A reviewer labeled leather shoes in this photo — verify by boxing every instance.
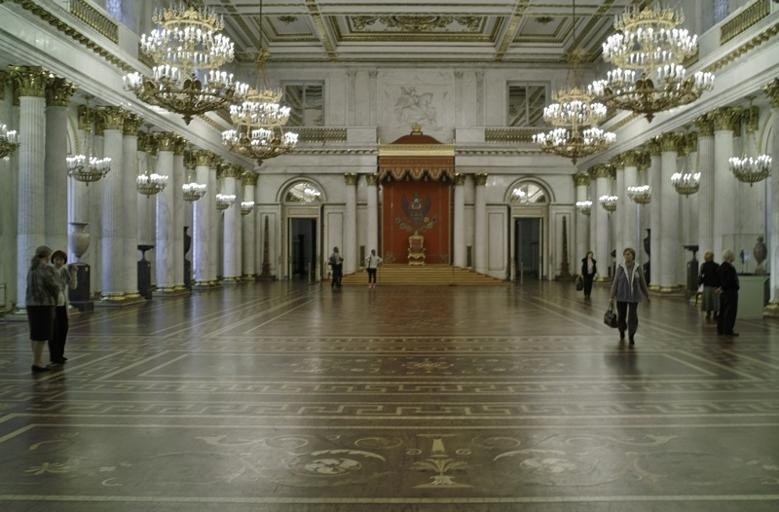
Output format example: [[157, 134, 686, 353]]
[[725, 332, 739, 336], [32, 356, 67, 372]]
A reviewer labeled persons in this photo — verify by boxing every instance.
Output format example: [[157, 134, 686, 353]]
[[328, 246, 344, 288], [44, 250, 79, 364], [25, 245, 63, 371], [363, 249, 383, 288], [608, 247, 653, 347], [713, 247, 740, 336], [580, 250, 597, 299], [697, 250, 721, 322]]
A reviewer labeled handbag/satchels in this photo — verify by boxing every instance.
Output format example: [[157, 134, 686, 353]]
[[603, 303, 617, 328], [575, 275, 584, 291]]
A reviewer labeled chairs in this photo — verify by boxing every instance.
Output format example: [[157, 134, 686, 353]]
[[406, 235, 426, 266]]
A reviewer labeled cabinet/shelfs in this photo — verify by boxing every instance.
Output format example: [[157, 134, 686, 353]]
[[735, 272, 770, 321]]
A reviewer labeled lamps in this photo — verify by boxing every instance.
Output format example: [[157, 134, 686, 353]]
[[0, 121, 24, 162], [121, 0, 300, 167], [531, 1, 717, 168], [64, 94, 256, 217], [573, 94, 772, 219]]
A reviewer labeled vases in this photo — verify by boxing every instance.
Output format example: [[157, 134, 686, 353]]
[[184, 226, 191, 256], [754, 237, 767, 265], [69, 221, 90, 265]]
